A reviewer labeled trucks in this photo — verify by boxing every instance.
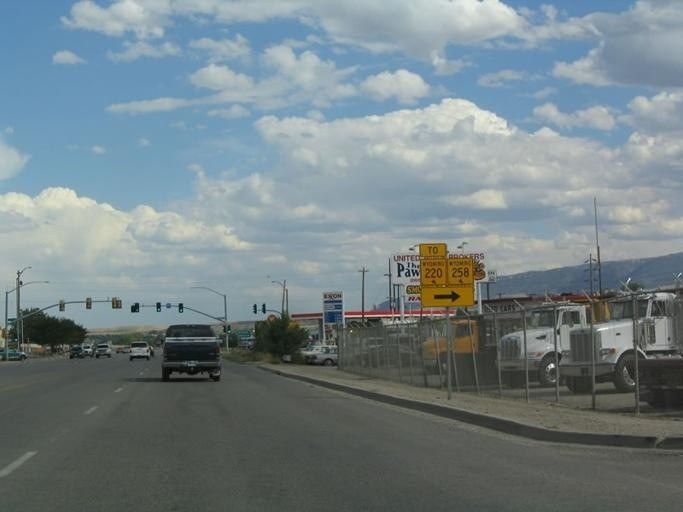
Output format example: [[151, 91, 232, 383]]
[[415, 310, 530, 374], [558, 291, 682, 393], [493, 302, 609, 387]]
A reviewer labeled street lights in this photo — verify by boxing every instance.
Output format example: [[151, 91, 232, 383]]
[[4, 280, 50, 362], [156, 302, 160, 312], [408, 243, 418, 250], [15, 266, 31, 355], [456, 240, 468, 254], [178, 302, 183, 313], [271, 280, 288, 322], [189, 286, 228, 351], [134, 302, 138, 312]]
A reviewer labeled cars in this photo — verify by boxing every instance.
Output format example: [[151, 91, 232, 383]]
[[237, 330, 419, 366], [68, 338, 154, 361], [0, 348, 29, 361]]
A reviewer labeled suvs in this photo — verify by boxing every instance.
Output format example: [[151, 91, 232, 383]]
[[161, 324, 220, 383]]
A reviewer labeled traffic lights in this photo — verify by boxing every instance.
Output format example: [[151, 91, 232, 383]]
[[227, 324, 229, 334], [262, 303, 265, 314], [253, 304, 256, 314], [222, 324, 225, 333]]
